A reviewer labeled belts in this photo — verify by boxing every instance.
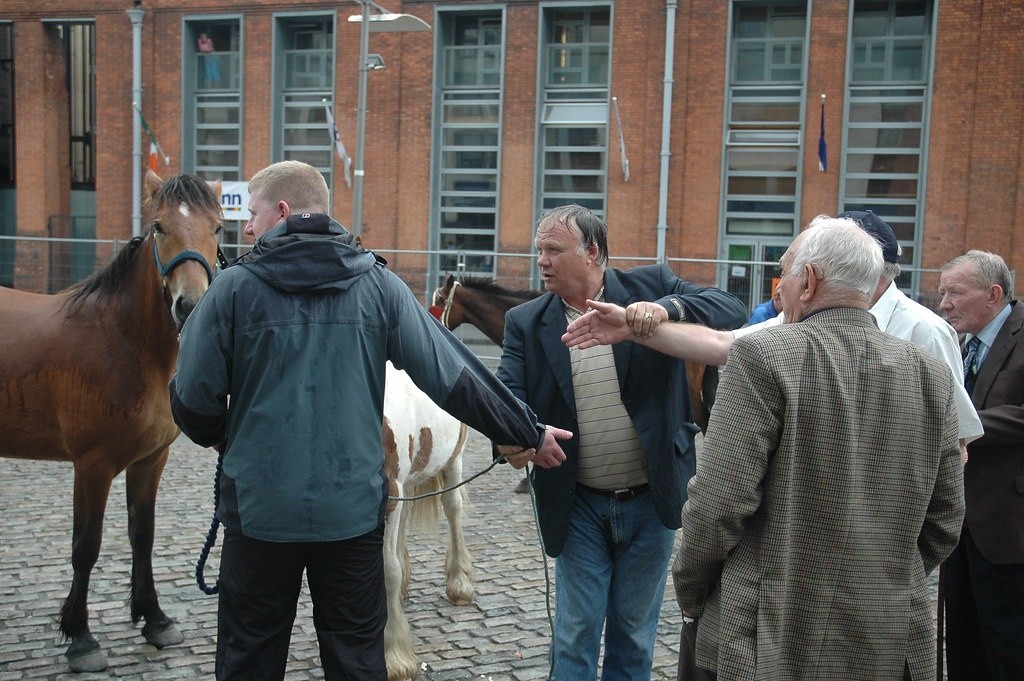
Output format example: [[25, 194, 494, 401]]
[[578, 482, 651, 502]]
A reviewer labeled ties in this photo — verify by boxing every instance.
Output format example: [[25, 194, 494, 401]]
[[963, 336, 982, 390]]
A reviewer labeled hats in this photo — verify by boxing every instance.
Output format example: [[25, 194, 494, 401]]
[[834, 211, 902, 263]]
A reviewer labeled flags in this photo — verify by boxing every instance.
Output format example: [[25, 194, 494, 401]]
[[139, 111, 159, 172], [322, 105, 352, 191], [613, 102, 628, 184], [817, 103, 829, 175]]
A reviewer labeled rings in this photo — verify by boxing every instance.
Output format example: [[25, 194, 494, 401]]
[[644, 313, 653, 319]]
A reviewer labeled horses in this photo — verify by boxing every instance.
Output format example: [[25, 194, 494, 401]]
[[0, 168, 224, 673], [381, 353, 474, 681], [427, 269, 721, 494]]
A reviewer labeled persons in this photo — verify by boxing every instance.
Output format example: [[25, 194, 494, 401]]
[[198, 32, 223, 88], [939, 247, 1024, 681], [492, 200, 749, 681], [167, 158, 573, 681], [561, 209, 985, 681]]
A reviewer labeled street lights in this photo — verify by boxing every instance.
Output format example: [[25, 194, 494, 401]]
[[343, 0, 431, 242]]
